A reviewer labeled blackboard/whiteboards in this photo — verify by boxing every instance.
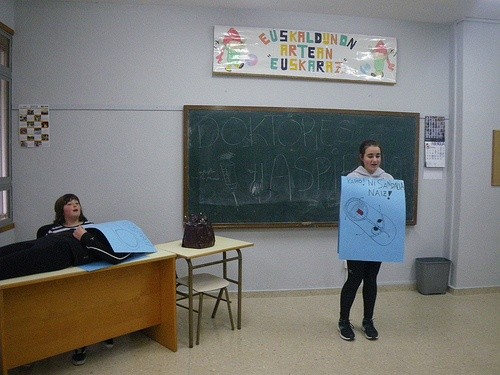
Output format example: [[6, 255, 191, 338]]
[[182, 105, 420, 229]]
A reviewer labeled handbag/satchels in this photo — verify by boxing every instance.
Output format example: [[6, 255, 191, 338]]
[[182, 213, 215, 249]]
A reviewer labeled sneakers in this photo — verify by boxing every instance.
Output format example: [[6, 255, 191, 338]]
[[103, 339, 114, 348], [361, 318, 379, 340], [336, 318, 356, 340], [72, 347, 87, 365]]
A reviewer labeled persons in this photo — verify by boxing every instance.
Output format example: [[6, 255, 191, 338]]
[[336, 140, 394, 341], [44, 194, 114, 364]]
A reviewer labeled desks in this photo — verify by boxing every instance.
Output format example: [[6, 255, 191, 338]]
[[154, 235, 254, 348], [0, 246, 177, 375]]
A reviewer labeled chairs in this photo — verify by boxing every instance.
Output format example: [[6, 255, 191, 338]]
[[176, 273, 234, 345]]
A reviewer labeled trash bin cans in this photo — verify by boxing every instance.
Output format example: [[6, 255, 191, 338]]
[[415, 257, 451, 295]]
